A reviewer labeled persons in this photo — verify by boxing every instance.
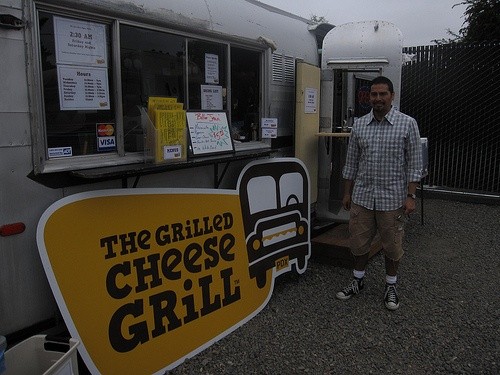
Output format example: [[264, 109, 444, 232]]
[[336, 75, 423, 309]]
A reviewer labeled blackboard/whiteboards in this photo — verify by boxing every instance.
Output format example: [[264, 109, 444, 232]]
[[185, 109, 236, 158]]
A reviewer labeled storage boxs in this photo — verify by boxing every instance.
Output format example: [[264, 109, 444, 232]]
[[5, 335, 80, 375]]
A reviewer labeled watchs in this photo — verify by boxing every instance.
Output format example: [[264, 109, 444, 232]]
[[408, 193, 416, 199]]
